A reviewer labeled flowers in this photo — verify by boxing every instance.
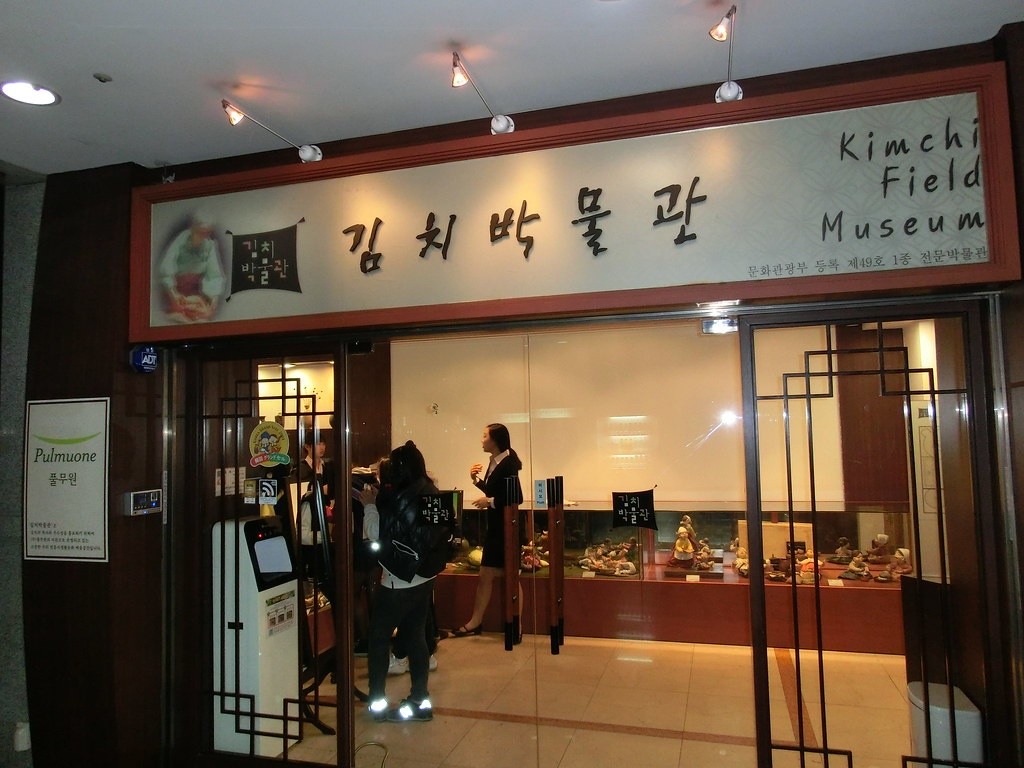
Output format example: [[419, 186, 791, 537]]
[[291, 384, 324, 404]]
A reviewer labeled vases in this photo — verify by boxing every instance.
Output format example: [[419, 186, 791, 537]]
[[303, 404, 311, 429]]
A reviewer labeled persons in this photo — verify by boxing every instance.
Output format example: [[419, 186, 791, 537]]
[[845, 550, 869, 578], [578, 537, 639, 576], [521, 527, 549, 573], [783, 545, 825, 584], [451, 424, 523, 644], [834, 533, 890, 564], [290, 431, 455, 722], [878, 548, 913, 580], [666, 515, 715, 570], [734, 546, 749, 575]]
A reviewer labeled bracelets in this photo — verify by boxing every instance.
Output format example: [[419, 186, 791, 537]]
[[486, 498, 490, 506]]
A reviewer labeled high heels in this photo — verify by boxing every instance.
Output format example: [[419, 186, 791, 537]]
[[451, 623, 482, 637], [519, 630, 522, 642]]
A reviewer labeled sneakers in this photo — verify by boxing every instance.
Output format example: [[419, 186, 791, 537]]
[[429, 654, 437, 671], [387, 698, 433, 721], [388, 658, 410, 673], [367, 696, 388, 722]]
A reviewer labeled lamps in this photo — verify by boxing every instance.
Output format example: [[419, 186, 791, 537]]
[[220, 98, 322, 164], [451, 51, 515, 134], [709, 4, 743, 103]]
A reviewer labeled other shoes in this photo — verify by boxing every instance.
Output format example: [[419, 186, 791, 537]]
[[433, 630, 448, 639]]
